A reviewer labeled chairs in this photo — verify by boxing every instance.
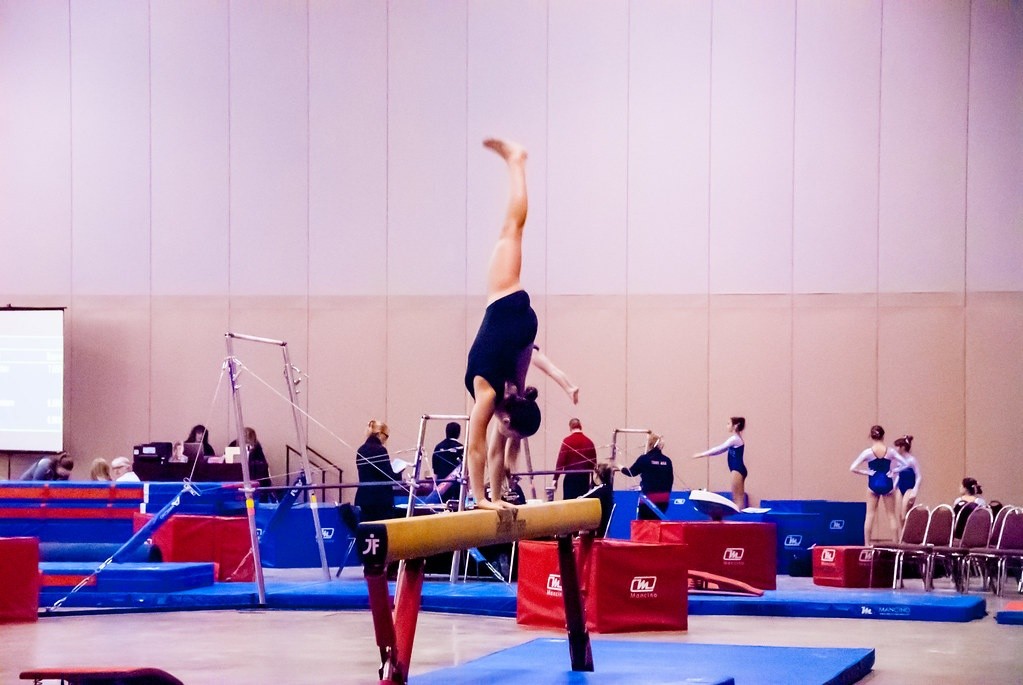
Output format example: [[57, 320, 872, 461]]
[[336, 502, 368, 577], [869, 498, 1023, 598]]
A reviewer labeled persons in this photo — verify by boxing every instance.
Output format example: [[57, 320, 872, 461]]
[[505, 345, 578, 470], [851, 425, 908, 547], [110, 457, 140, 482], [551, 418, 597, 501], [953, 478, 984, 507], [243, 427, 272, 504], [186, 425, 215, 456], [355, 420, 404, 521], [613, 434, 672, 521], [893, 436, 921, 539], [576, 464, 612, 537], [21, 452, 73, 481], [692, 417, 747, 511], [464, 139, 537, 510], [431, 422, 469, 505], [167, 441, 188, 463], [89, 458, 110, 481]]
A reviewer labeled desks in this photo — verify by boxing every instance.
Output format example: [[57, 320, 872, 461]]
[[387, 504, 511, 578]]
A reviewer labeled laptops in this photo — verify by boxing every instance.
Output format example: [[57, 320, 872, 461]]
[[225, 447, 248, 463], [183, 443, 206, 464]]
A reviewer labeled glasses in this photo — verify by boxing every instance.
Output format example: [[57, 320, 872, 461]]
[[381, 431, 389, 438], [112, 466, 124, 471]]
